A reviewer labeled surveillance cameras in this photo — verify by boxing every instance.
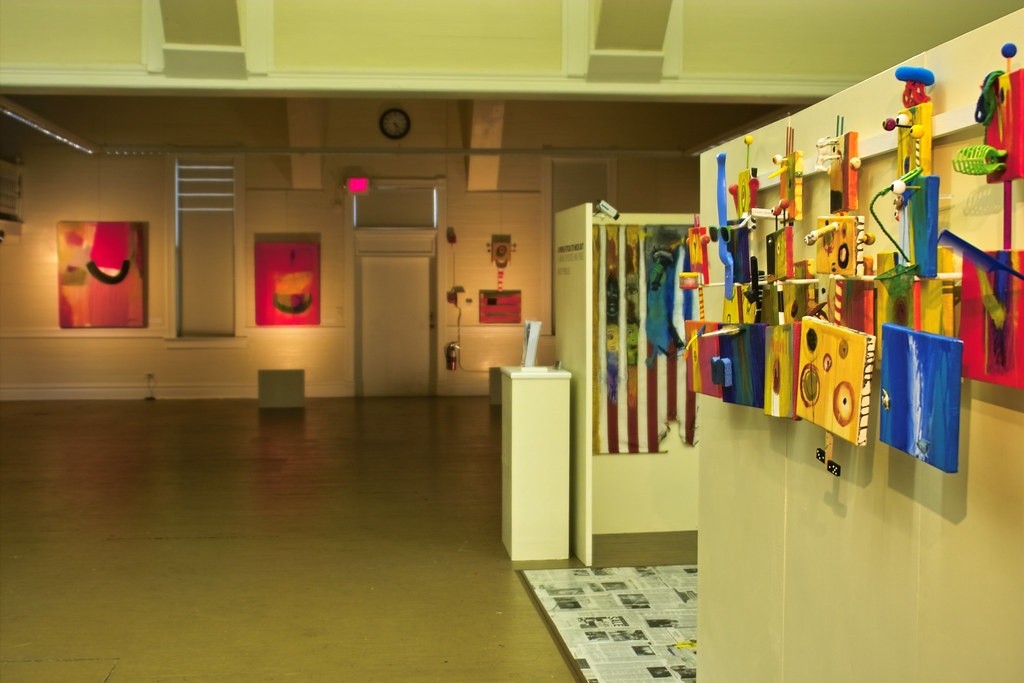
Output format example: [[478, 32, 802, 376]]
[[597, 200, 620, 220]]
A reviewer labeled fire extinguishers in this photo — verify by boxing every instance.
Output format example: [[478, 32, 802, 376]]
[[447, 341, 460, 370]]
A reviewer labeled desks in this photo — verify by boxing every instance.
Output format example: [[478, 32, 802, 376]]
[[499, 364, 573, 570]]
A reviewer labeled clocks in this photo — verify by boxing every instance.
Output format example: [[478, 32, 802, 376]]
[[379, 108, 410, 139]]
